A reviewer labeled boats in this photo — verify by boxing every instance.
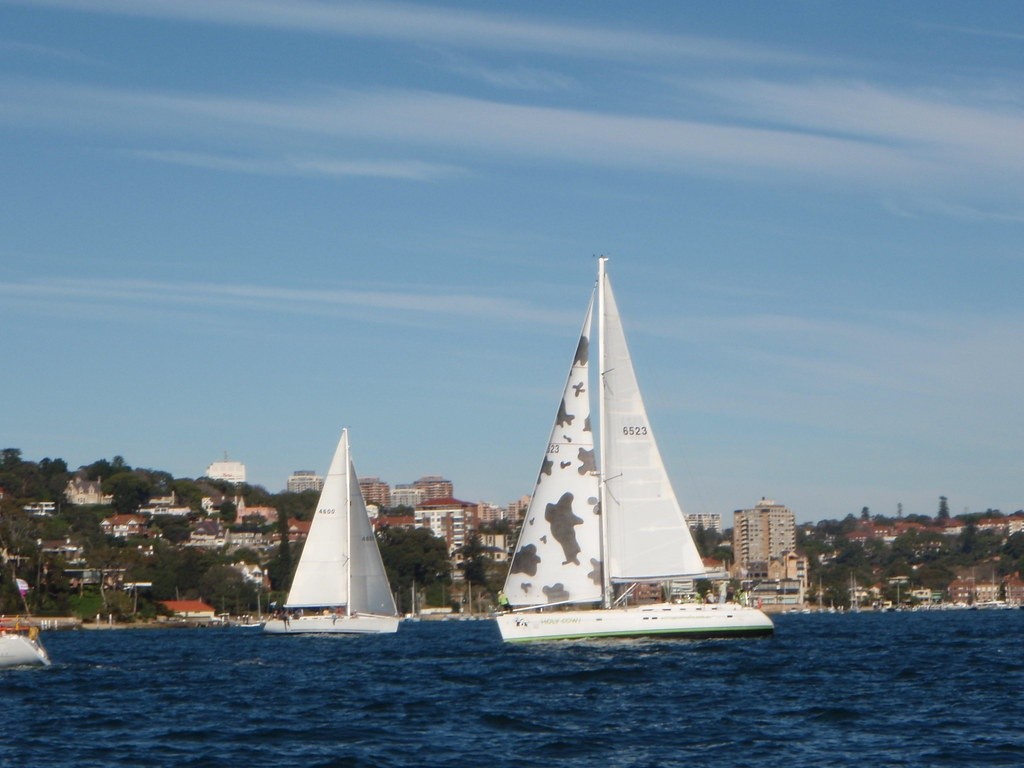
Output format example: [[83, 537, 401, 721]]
[[0, 532, 51, 671]]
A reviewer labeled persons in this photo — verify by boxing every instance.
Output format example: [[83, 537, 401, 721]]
[[498, 591, 509, 611], [273, 607, 357, 631], [705, 587, 743, 605]]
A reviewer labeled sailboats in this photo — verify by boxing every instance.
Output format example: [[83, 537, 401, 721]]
[[488, 252, 774, 643], [262, 427, 401, 634], [780, 566, 1024, 614], [408, 578, 489, 621]]
